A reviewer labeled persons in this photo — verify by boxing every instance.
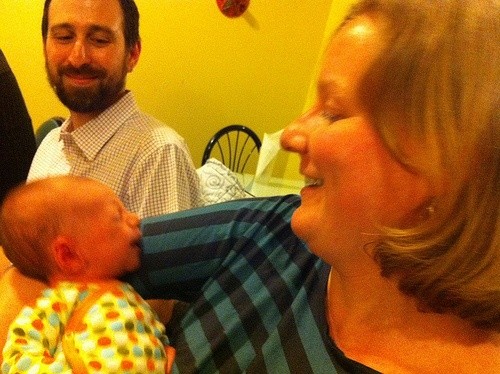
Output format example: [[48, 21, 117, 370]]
[[0, 0, 500, 374], [25, 0, 203, 219], [0, 177, 177, 374]]
